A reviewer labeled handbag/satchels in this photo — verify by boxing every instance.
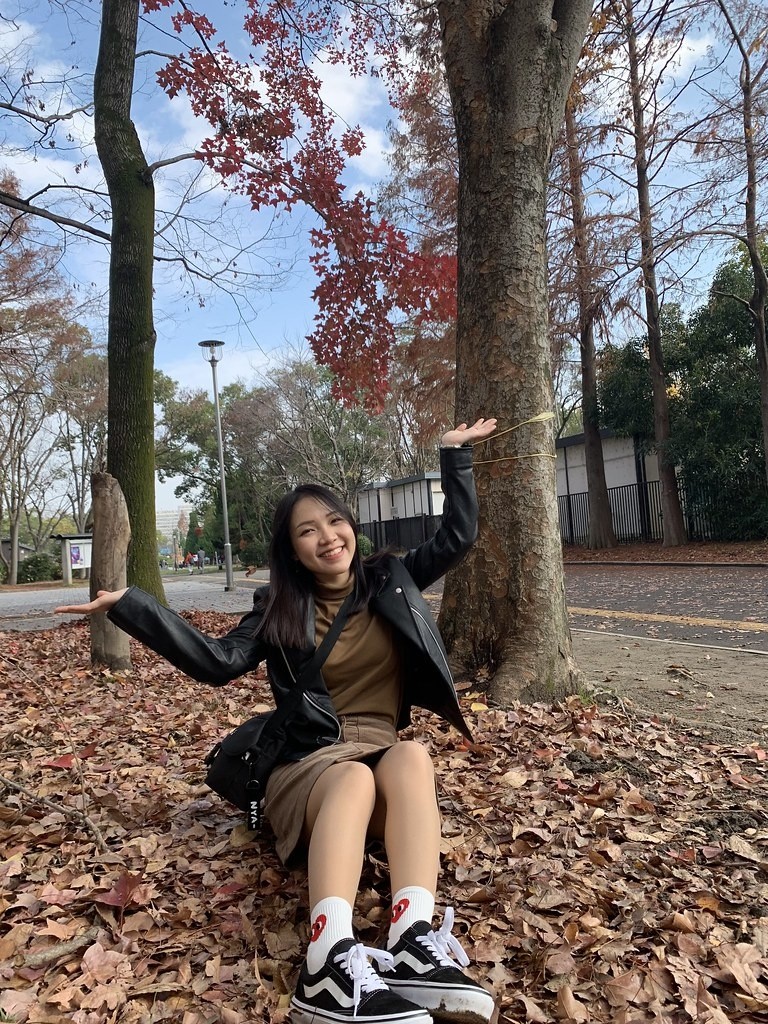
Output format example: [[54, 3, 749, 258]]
[[203, 706, 296, 831]]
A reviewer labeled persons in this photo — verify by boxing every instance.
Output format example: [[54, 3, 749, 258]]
[[197, 547, 205, 574], [54, 418, 497, 1024], [185, 551, 193, 575]]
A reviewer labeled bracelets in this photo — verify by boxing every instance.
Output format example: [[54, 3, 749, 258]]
[[440, 445, 460, 448]]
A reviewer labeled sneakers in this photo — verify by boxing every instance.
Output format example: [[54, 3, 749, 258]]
[[371, 906, 495, 1024], [289, 938, 433, 1024]]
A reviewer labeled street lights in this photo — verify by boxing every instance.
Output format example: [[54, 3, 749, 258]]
[[198, 340, 235, 592]]
